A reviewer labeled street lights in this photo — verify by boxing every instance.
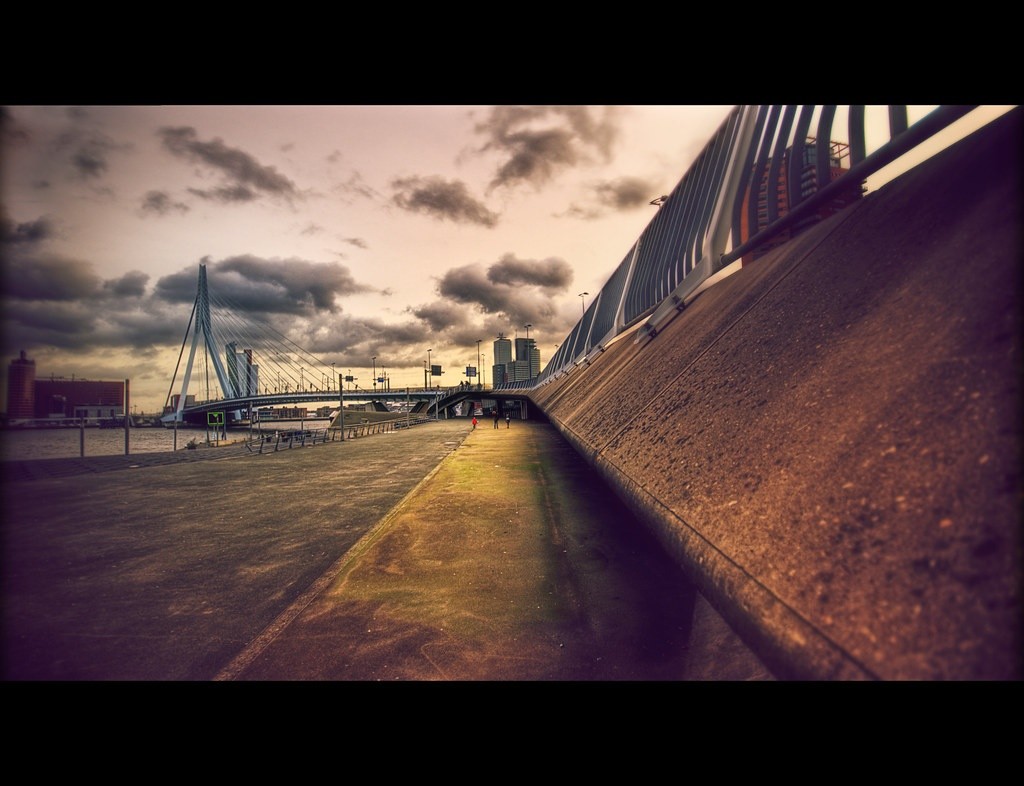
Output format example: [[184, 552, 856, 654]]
[[476, 339, 486, 391], [257, 367, 306, 392], [329, 363, 336, 392], [424, 349, 434, 390], [372, 356, 378, 391], [580, 292, 589, 313], [523, 323, 532, 379]]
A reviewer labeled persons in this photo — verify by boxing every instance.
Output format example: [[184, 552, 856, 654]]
[[505, 412, 510, 428], [472, 416, 478, 429], [493, 405, 499, 429]]
